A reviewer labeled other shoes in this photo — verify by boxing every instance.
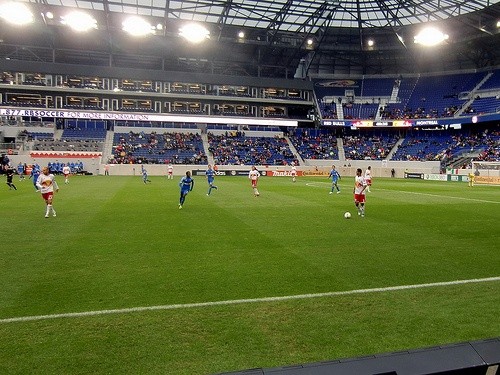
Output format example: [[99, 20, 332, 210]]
[[329, 192, 332, 194], [215, 186, 218, 190], [337, 191, 340, 194], [255, 192, 259, 196], [357, 211, 365, 218], [178, 204, 182, 210], [206, 194, 209, 196]]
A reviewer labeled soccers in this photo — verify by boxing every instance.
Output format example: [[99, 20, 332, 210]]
[[345, 212, 351, 218]]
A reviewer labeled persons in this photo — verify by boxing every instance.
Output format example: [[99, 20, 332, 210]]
[[35, 126, 101, 153], [353, 169, 367, 217], [179, 171, 194, 209], [328, 165, 342, 194], [3, 148, 17, 190], [365, 166, 372, 192], [167, 164, 173, 179], [37, 166, 59, 218], [140, 165, 151, 184], [322, 105, 474, 119], [286, 129, 500, 178], [105, 164, 109, 175], [249, 166, 260, 195], [467, 172, 475, 187], [18, 162, 40, 191], [48, 161, 83, 183], [108, 130, 299, 166], [291, 168, 296, 181], [206, 164, 218, 196]]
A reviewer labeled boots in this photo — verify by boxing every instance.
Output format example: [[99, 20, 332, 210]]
[[51, 205, 56, 218], [44, 205, 52, 217]]
